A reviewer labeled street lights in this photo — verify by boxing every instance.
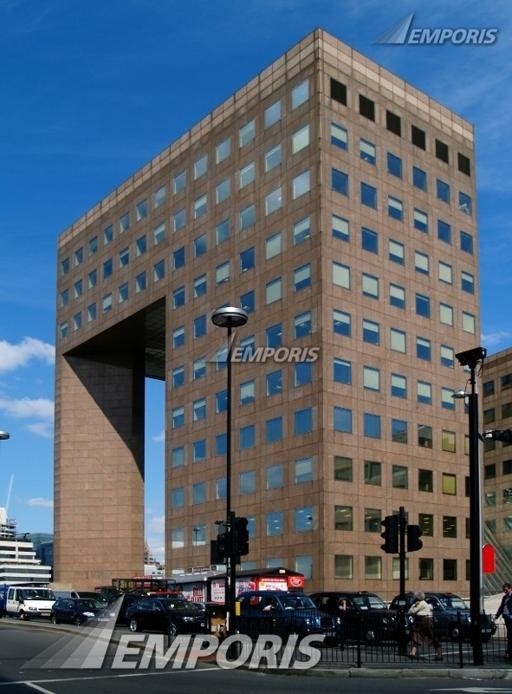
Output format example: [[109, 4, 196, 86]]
[[210, 306, 249, 656], [449, 388, 483, 664]]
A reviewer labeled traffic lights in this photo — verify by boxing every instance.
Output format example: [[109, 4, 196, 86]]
[[379, 515, 397, 554], [407, 524, 423, 552], [230, 517, 249, 557], [216, 531, 231, 556]]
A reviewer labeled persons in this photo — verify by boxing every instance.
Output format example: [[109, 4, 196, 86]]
[[407, 590, 444, 660], [493, 581, 512, 662]]
